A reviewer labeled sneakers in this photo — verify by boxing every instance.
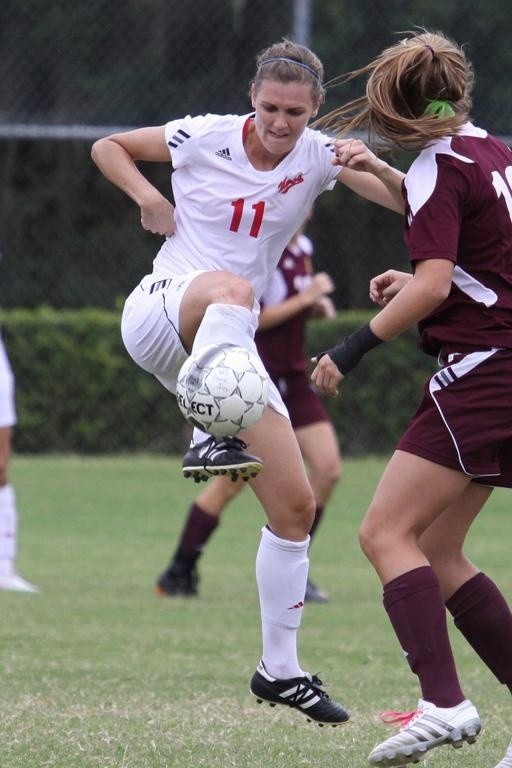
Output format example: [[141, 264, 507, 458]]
[[365, 695, 485, 768], [250, 657, 351, 728], [154, 566, 201, 598], [181, 434, 264, 483]]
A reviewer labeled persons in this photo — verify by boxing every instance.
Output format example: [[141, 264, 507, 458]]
[[154, 206, 343, 604], [2, 340, 38, 595], [91, 36, 410, 729], [309, 21, 512, 768]]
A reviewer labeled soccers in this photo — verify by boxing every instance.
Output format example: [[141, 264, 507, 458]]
[[175, 342, 270, 439]]
